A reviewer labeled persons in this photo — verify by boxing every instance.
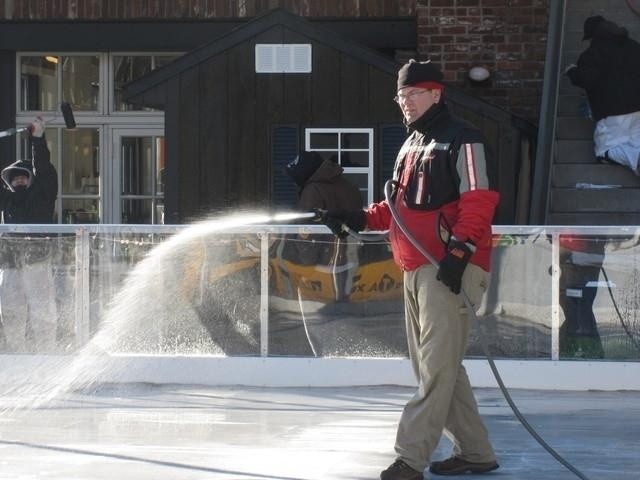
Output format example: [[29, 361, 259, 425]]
[[285, 151, 364, 267], [322, 57, 502, 480], [564, 14, 639, 173], [546, 234, 607, 360], [0, 114, 60, 355]]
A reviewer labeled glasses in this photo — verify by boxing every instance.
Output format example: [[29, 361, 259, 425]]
[[393, 90, 430, 104]]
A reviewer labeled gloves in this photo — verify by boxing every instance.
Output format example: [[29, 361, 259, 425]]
[[321, 210, 366, 238], [436, 234, 477, 294]]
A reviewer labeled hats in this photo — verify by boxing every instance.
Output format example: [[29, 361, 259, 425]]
[[397, 59, 444, 91], [581, 16, 605, 41]]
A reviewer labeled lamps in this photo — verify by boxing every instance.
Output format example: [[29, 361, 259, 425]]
[[467, 59, 493, 83]]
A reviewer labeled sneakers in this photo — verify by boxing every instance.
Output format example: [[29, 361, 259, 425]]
[[381, 460, 424, 480], [430, 457, 499, 475]]
